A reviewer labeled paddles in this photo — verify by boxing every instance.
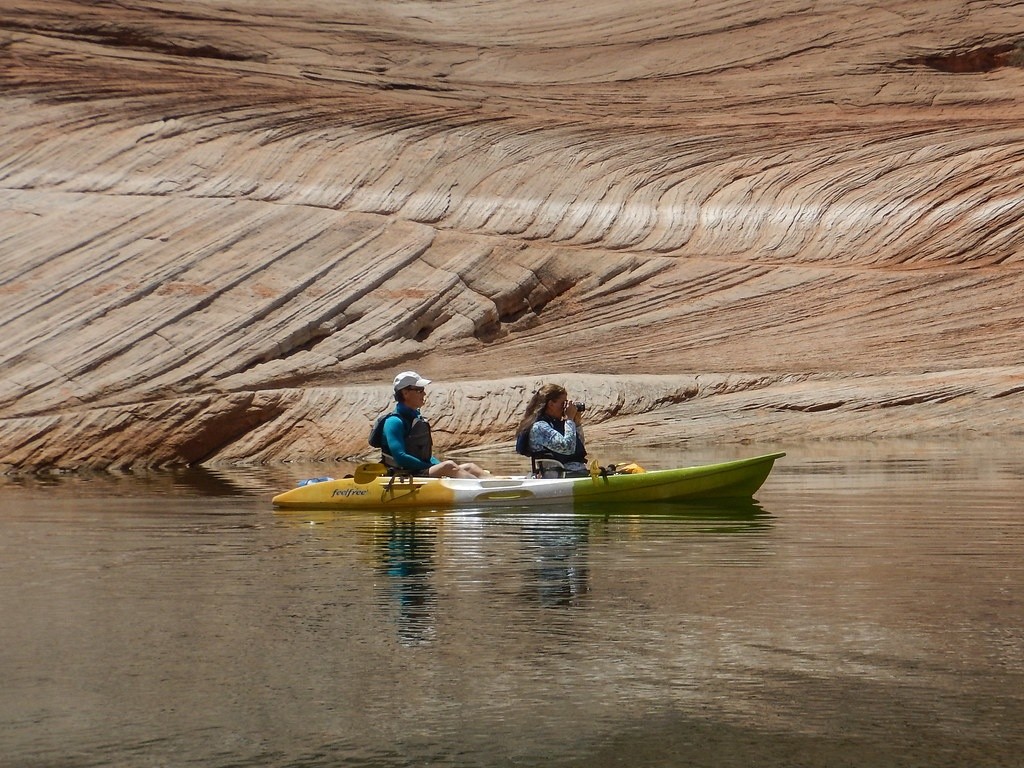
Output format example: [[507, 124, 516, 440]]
[[353, 462, 413, 484], [589, 458, 629, 483]]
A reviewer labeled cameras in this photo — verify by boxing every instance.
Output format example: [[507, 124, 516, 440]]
[[565, 400, 585, 412]]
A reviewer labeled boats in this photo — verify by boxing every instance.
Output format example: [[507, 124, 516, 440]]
[[271, 450, 785, 510]]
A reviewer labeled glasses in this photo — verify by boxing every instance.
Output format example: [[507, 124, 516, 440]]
[[403, 386, 424, 394]]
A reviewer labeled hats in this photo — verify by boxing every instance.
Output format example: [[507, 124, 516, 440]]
[[393, 370, 431, 393]]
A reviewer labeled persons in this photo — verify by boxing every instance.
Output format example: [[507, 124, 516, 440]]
[[513, 383, 590, 476], [380, 371, 512, 479]]
